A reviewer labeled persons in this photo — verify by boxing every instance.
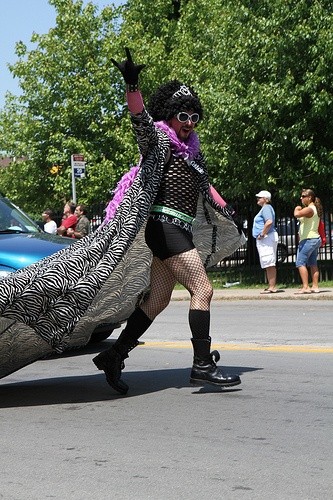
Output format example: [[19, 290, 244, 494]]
[[93, 46, 247, 396], [43, 200, 91, 240], [252, 190, 279, 294], [294, 189, 322, 294]]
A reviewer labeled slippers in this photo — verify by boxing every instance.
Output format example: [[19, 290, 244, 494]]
[[294, 289, 311, 294], [310, 289, 320, 293], [261, 290, 277, 294]]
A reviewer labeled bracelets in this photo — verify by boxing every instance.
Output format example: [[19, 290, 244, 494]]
[[259, 234, 264, 237], [126, 84, 139, 92]]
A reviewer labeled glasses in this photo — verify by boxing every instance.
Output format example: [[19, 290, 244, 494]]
[[301, 195, 309, 198], [176, 111, 199, 123]]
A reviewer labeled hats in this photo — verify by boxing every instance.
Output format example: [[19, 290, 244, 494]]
[[255, 190, 272, 199]]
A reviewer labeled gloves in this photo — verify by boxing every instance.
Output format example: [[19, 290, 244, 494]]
[[110, 47, 146, 85], [232, 212, 249, 238]]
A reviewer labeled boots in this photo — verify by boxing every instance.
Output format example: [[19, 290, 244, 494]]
[[91, 330, 139, 395], [189, 336, 241, 387]]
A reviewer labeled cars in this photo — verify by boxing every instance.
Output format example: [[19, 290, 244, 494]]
[[0, 194, 128, 354], [216, 220, 299, 265]]
